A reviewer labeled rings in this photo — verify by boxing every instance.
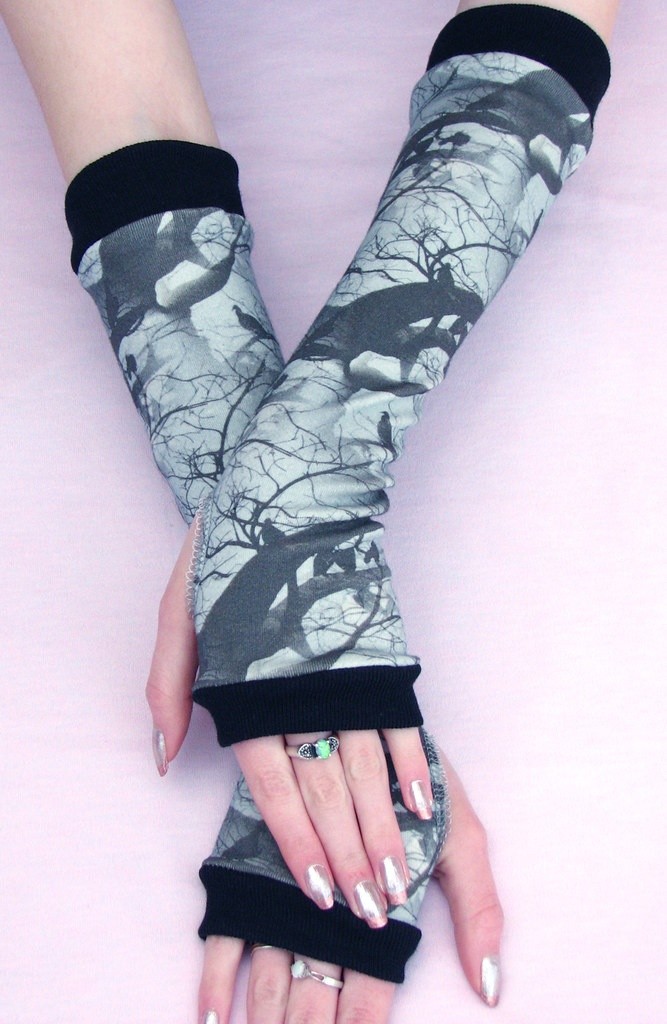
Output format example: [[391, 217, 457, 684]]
[[289, 960, 344, 989], [249, 943, 272, 957], [284, 735, 339, 761]]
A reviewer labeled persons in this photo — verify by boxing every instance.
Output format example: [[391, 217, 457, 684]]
[[0, 0, 620, 1024]]
